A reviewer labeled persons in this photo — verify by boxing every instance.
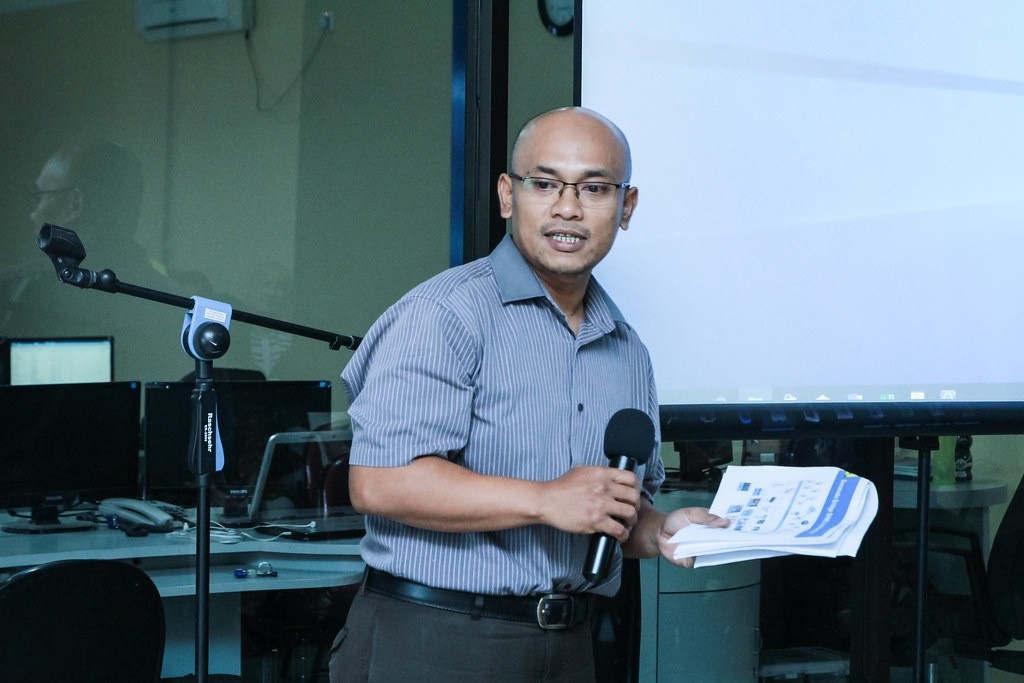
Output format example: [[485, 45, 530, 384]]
[[328, 106, 879, 683]]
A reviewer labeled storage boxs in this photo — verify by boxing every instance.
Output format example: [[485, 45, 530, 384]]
[[759, 646, 850, 683]]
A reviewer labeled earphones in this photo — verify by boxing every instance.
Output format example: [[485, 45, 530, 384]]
[[309, 521, 316, 528]]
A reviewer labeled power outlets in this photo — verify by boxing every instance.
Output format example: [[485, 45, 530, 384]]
[[318, 10, 335, 32]]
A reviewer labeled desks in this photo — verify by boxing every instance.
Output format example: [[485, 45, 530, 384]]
[[893, 476, 1008, 683], [1, 488, 763, 683]]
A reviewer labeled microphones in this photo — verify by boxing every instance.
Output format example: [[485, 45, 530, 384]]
[[583, 408, 655, 583]]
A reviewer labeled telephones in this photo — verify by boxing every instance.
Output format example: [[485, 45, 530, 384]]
[[98, 498, 198, 532]]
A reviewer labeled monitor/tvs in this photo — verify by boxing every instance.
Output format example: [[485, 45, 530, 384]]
[[0, 337, 368, 534]]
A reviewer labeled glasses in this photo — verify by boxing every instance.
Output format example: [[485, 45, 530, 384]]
[[508, 173, 630, 209]]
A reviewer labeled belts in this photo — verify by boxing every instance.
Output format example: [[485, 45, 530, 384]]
[[362, 565, 594, 630]]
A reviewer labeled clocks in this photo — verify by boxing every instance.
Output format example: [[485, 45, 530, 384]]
[[537, 0, 574, 37]]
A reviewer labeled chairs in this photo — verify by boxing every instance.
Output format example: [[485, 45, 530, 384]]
[[891, 475, 1024, 683], [0, 559, 167, 683]]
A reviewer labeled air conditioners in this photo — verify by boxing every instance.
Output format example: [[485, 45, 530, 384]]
[[135, 0, 255, 44]]
